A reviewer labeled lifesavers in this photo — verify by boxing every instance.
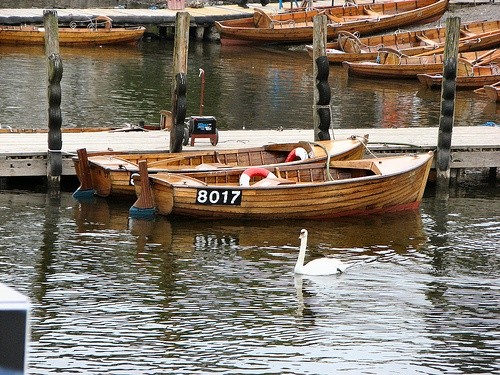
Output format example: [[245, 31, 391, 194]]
[[240, 167, 278, 187], [285, 147, 309, 163]]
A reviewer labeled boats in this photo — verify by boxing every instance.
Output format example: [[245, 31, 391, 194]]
[[213, 1, 448, 46], [0, 15, 147, 46], [72, 133, 434, 220], [303, 19, 500, 100]]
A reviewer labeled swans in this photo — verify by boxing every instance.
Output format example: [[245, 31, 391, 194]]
[[294, 228, 355, 275]]
